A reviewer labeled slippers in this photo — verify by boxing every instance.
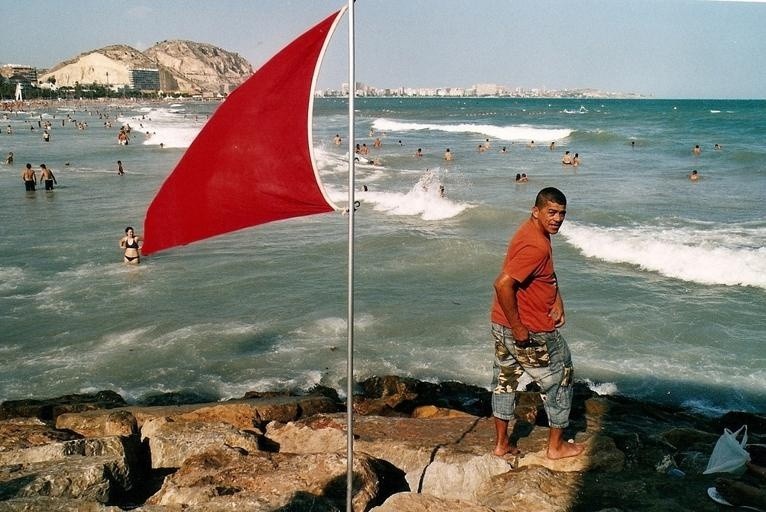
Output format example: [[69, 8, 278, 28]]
[[708, 486, 761, 512]]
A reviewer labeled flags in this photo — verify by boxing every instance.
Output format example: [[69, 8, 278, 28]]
[[142, 5, 348, 256]]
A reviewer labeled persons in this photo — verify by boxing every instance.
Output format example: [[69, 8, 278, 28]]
[[488, 187, 587, 459], [689, 169, 700, 181], [119, 226, 144, 266], [334, 127, 582, 198], [0, 95, 226, 191], [631, 141, 635, 145], [694, 144, 700, 154], [715, 144, 722, 151]]
